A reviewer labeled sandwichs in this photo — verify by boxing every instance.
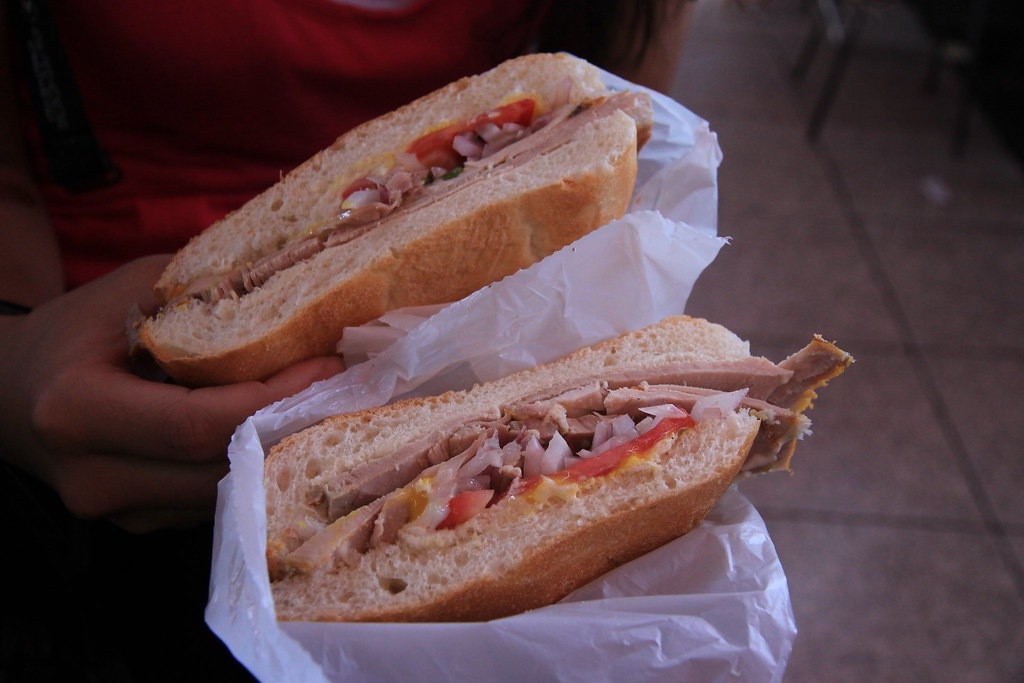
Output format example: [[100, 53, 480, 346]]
[[135, 47, 652, 380], [265, 316, 849, 628]]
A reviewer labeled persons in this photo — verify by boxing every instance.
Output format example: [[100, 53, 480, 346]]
[[0, 0, 696, 683]]
